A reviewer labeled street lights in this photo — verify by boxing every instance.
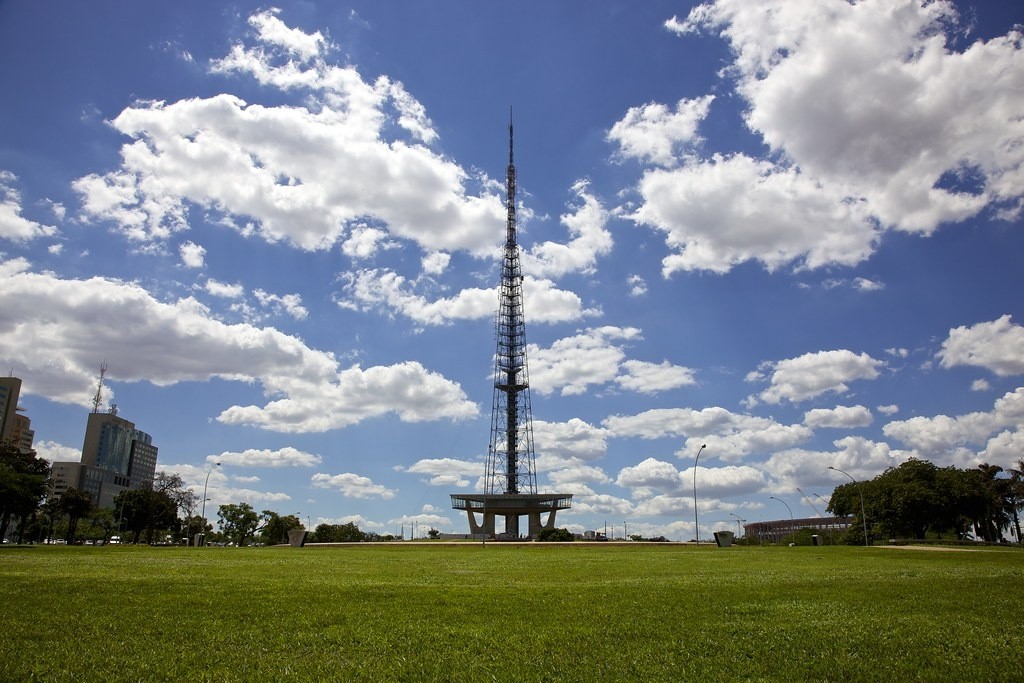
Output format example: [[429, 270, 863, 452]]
[[730, 512, 746, 523], [769, 495, 796, 544], [186, 497, 211, 545], [828, 465, 868, 546], [199, 463, 222, 544], [693, 444, 707, 542]]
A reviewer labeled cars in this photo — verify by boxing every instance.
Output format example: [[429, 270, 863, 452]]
[[41, 536, 103, 545]]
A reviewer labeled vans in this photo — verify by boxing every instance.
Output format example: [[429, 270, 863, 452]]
[[109, 535, 122, 542]]
